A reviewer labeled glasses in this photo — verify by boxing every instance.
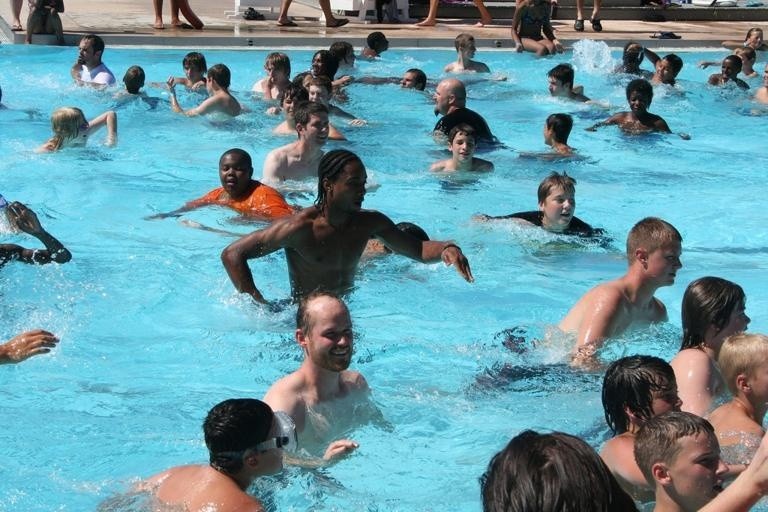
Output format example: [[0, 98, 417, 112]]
[[208, 411, 298, 461]]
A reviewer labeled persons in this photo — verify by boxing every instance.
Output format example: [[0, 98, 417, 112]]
[[1, 329, 61, 364], [71, 34, 116, 84], [276, 1, 349, 27], [543, 113, 574, 153], [491, 171, 593, 236], [576, 1, 601, 32], [557, 214, 685, 348], [263, 285, 394, 470], [699, 28, 768, 104], [400, 68, 427, 92], [220, 150, 474, 312], [417, 1, 490, 27], [152, 1, 185, 28], [605, 79, 670, 133], [10, 1, 65, 47], [547, 63, 589, 102], [433, 33, 495, 171], [259, 100, 379, 199], [641, 1, 665, 8], [252, 32, 391, 143], [45, 107, 119, 153], [123, 51, 241, 116], [617, 44, 683, 83], [375, 1, 400, 24], [0, 192, 72, 266], [146, 148, 296, 220], [96, 397, 286, 512], [512, 1, 561, 57], [480, 276, 768, 511]]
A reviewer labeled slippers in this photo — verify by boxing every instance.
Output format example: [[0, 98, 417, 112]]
[[649, 31, 681, 39], [589, 17, 602, 31], [326, 19, 348, 27], [10, 24, 21, 31], [277, 22, 297, 26], [573, 18, 584, 31]]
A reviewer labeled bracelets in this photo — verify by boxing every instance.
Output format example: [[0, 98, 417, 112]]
[[442, 244, 464, 251]]
